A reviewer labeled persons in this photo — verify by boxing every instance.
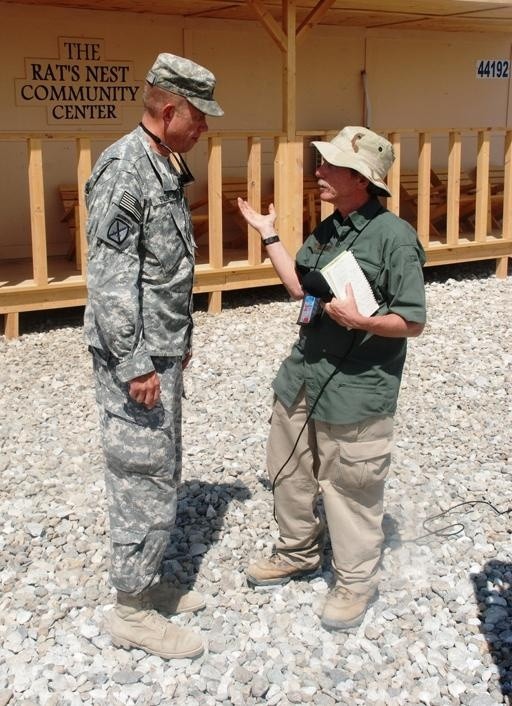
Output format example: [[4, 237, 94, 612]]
[[82, 49, 229, 662], [234, 123, 431, 635]]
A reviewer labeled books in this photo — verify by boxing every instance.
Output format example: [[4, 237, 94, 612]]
[[318, 249, 382, 318]]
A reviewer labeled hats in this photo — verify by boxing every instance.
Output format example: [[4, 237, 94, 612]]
[[143, 51, 227, 117], [308, 123, 396, 198]]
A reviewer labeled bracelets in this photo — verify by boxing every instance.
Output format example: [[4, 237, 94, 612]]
[[261, 234, 280, 248]]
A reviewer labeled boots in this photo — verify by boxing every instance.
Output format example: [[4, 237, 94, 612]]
[[108, 579, 208, 660]]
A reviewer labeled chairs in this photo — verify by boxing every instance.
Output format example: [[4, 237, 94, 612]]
[[57, 165, 509, 272]]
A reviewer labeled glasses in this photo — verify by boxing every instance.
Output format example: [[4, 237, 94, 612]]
[[158, 141, 196, 187]]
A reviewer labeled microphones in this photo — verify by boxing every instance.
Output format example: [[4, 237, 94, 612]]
[[301, 271, 353, 333]]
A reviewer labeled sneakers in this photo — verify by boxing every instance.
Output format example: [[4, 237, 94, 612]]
[[244, 549, 325, 586], [320, 583, 381, 630]]
